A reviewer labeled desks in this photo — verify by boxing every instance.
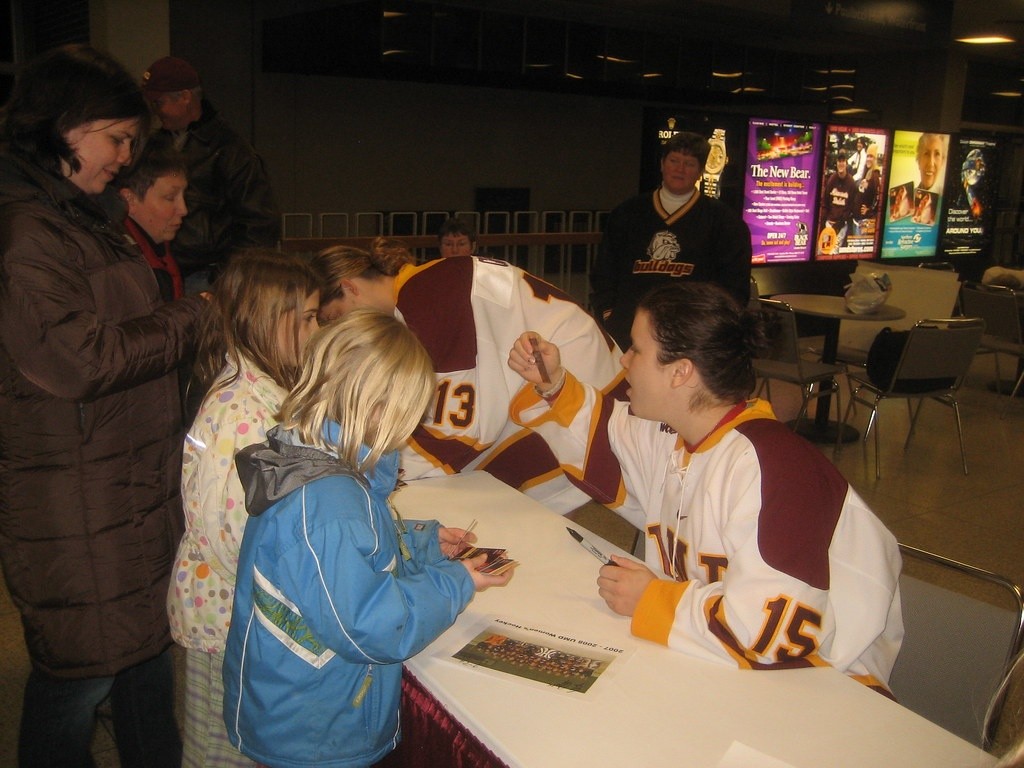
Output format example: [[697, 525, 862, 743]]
[[385, 438, 1024, 768], [760, 290, 907, 445]]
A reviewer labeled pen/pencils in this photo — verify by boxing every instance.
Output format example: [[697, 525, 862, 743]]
[[566, 527, 619, 566]]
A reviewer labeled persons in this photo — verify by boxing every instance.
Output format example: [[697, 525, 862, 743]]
[[888, 132, 946, 226], [820, 137, 880, 247], [507, 282, 904, 704], [303, 216, 624, 491], [223, 308, 518, 768], [587, 132, 752, 353], [166, 246, 322, 768], [120, 56, 276, 308], [0, 46, 223, 768]]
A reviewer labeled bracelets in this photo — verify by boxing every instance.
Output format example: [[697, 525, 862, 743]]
[[531, 367, 567, 398]]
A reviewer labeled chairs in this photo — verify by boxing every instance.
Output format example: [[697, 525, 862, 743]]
[[947, 282, 1024, 420], [847, 314, 987, 480], [753, 297, 841, 445], [888, 544, 1024, 757]]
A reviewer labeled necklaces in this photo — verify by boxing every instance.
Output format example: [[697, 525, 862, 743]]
[[658, 431, 698, 577]]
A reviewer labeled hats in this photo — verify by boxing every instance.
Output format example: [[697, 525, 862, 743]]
[[138, 56, 201, 98]]
